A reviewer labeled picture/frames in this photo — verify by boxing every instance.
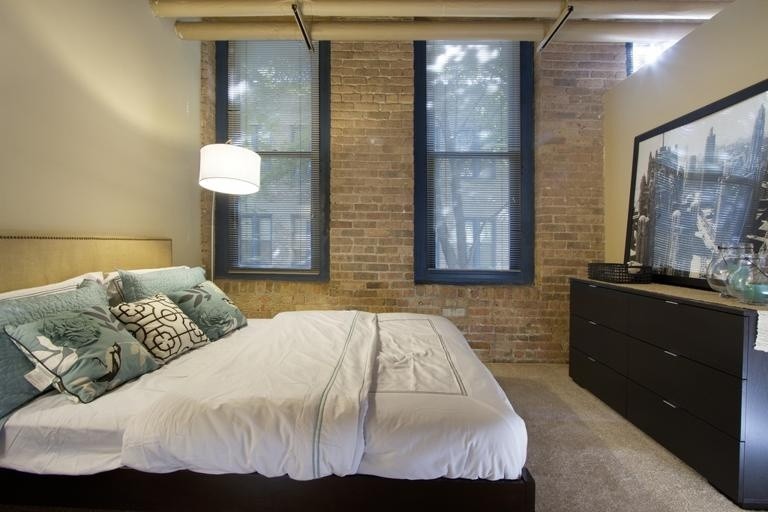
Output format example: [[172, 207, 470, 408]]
[[622, 79, 767, 293]]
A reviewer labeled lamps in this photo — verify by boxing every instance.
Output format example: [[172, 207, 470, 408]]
[[196, 140, 262, 284]]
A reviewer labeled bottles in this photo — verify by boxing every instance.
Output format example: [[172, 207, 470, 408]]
[[707, 243, 754, 298], [728, 254, 768, 305]]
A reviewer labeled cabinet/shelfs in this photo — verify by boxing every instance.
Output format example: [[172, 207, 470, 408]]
[[625, 289, 768, 512], [565, 275, 634, 425]]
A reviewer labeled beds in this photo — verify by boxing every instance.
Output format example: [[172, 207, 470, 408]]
[[0, 235, 537, 512]]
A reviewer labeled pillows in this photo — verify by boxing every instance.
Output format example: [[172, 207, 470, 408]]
[[109, 291, 210, 364], [167, 279, 248, 342], [115, 265, 206, 303], [0, 281, 109, 419], [0, 271, 106, 300], [105, 267, 189, 308], [5, 305, 162, 404]]
